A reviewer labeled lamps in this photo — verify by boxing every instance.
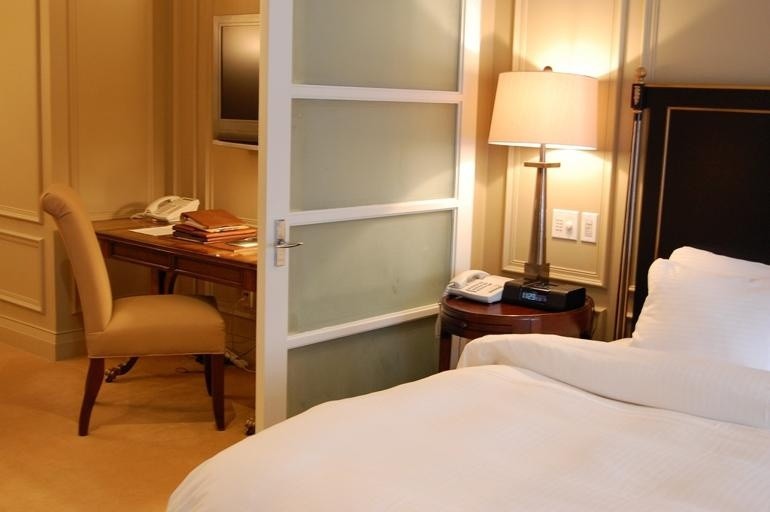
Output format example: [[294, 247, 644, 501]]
[[487, 67, 598, 282]]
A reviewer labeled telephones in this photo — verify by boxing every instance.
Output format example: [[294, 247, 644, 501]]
[[143, 195, 200, 222], [446, 269, 515, 304]]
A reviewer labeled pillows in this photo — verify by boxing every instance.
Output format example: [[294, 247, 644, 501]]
[[632, 257, 770, 370], [669, 246, 770, 279]]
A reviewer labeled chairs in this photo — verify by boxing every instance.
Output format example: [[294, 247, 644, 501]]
[[39, 183, 227, 437]]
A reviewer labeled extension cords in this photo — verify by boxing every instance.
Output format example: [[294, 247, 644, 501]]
[[224, 348, 248, 369]]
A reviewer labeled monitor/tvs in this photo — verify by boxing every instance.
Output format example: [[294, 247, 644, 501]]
[[211, 13, 261, 144]]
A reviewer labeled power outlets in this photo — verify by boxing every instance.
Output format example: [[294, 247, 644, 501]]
[[240, 292, 254, 309]]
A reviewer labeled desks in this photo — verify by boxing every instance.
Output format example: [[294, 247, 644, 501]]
[[438, 296, 594, 370], [93, 215, 258, 383]]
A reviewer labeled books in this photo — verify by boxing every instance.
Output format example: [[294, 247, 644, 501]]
[[173, 210, 257, 243]]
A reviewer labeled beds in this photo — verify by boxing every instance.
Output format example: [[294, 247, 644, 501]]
[[168, 84, 770, 512]]
